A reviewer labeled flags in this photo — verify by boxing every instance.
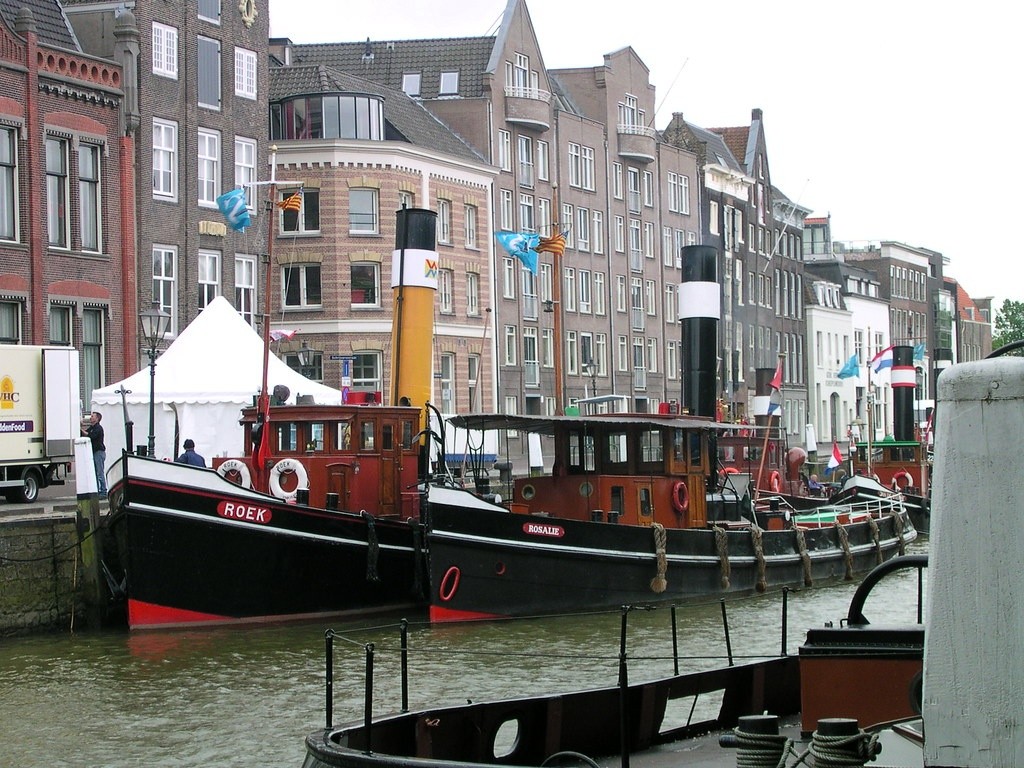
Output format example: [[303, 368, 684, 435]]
[[529, 231, 569, 255], [825, 438, 842, 474], [872, 345, 895, 374], [913, 343, 924, 360], [837, 355, 859, 379], [276, 186, 302, 210], [216, 188, 252, 233], [768, 361, 783, 419], [270, 329, 298, 341], [495, 231, 538, 277]]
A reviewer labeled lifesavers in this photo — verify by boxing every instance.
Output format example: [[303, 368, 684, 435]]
[[720, 467, 739, 476], [216, 459, 251, 491], [269, 459, 308, 502], [892, 472, 913, 492], [674, 482, 688, 512], [770, 471, 781, 493]]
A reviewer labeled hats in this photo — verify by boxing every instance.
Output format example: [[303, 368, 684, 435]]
[[183, 439, 195, 449]]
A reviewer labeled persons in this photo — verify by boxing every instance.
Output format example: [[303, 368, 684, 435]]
[[809, 474, 827, 497], [175, 439, 206, 467], [81, 411, 108, 500]]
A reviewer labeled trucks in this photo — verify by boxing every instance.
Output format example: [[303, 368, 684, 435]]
[[429, 412, 498, 477], [0, 344, 82, 502]]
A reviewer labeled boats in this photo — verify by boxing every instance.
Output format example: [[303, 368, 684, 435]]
[[423, 182, 906, 622], [97, 383, 430, 630], [304, 556, 929, 768], [713, 424, 934, 538]]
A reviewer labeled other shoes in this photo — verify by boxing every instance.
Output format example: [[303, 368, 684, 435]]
[[99, 496, 107, 500]]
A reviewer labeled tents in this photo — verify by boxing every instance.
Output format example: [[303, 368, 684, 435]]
[[93, 296, 438, 499]]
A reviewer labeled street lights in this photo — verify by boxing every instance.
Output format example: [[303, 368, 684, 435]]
[[585, 358, 601, 414], [296, 341, 315, 378], [870, 381, 877, 442], [140, 301, 172, 458]]
